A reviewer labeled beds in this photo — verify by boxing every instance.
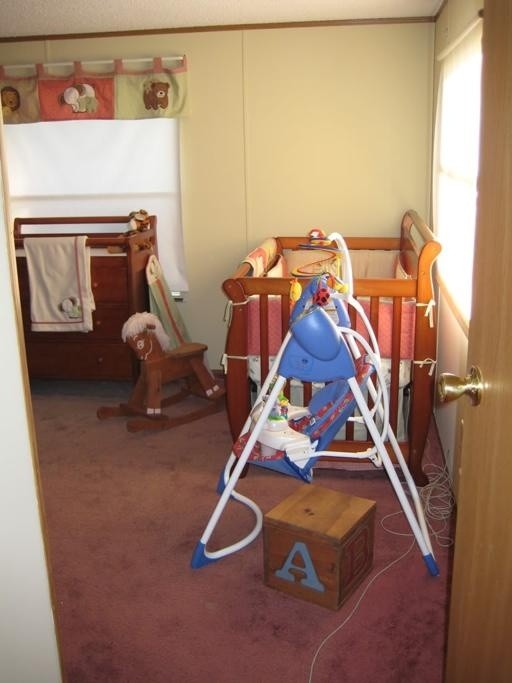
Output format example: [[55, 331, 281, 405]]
[[220, 209, 443, 486]]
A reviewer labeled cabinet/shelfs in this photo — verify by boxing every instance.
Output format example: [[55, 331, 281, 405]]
[[14, 216, 158, 382]]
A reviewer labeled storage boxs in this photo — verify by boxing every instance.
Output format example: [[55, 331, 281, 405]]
[[263, 484, 376, 611]]
